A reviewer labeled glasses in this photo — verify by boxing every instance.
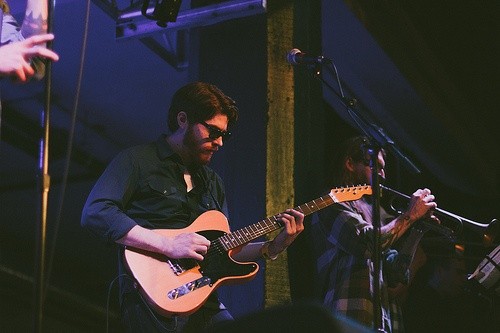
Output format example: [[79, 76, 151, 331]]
[[357, 157, 389, 172], [195, 118, 232, 140]]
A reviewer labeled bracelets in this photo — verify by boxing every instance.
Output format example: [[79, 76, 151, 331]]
[[260, 240, 280, 262]]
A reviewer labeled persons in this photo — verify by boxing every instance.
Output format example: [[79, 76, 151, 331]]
[[0, 0, 60, 85], [310, 135, 442, 333], [79, 80, 305, 333]]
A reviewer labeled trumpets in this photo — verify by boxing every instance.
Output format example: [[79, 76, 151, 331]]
[[378, 183, 498, 248]]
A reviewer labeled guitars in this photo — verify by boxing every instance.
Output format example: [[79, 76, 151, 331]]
[[122, 183, 373, 314]]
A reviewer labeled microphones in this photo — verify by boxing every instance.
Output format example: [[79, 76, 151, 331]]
[[287, 48, 326, 65]]
